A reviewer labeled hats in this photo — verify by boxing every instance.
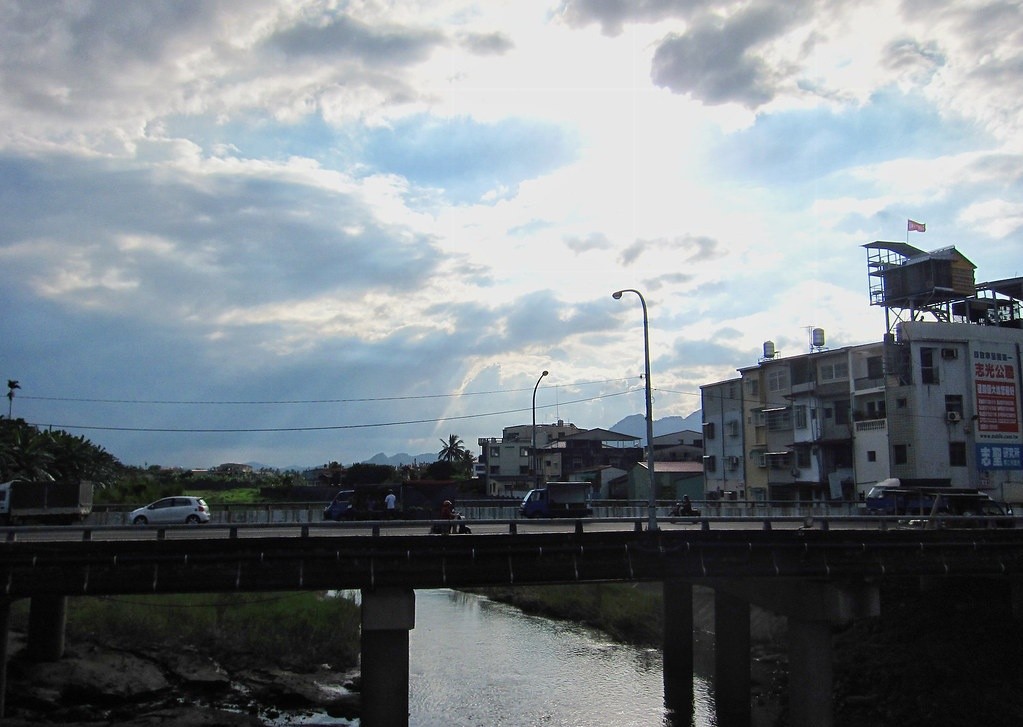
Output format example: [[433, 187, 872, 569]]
[[388, 489, 392, 491]]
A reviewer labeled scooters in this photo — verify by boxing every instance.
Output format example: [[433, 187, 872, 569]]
[[667, 504, 701, 525], [430, 509, 471, 534]]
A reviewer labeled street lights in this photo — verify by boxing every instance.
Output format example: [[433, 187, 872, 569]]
[[532, 370, 549, 487], [612, 288, 663, 531]]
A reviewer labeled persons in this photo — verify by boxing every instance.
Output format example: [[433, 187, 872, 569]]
[[440, 500, 459, 534], [384, 489, 396, 520], [679, 495, 691, 517]]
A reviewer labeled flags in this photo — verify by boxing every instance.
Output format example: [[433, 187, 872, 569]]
[[908, 219, 926, 232]]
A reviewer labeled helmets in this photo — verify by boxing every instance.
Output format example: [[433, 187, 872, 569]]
[[684, 496, 688, 500], [444, 500, 452, 505]]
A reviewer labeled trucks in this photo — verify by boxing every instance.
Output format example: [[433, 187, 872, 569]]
[[859, 477, 989, 518], [0, 477, 94, 527], [321, 481, 457, 521], [518, 481, 595, 518]]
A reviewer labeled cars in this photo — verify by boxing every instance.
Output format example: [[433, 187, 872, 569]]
[[127, 496, 210, 530]]
